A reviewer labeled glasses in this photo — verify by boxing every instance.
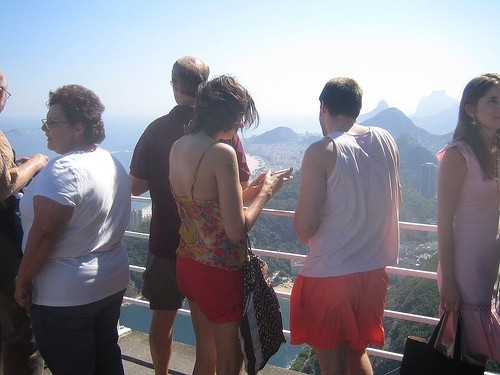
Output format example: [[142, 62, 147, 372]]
[[41, 118, 68, 129], [0, 86, 12, 99]]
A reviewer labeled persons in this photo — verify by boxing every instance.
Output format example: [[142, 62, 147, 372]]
[[14, 84, 131, 375], [129, 55, 250, 375], [436, 73, 500, 375], [168, 74, 294, 375], [290, 77, 402, 375], [0, 72, 49, 375]]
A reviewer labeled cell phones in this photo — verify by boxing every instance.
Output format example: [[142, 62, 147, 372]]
[[270, 169, 289, 178]]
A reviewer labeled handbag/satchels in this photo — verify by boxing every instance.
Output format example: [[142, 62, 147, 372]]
[[398, 308, 490, 375], [236, 214, 288, 375]]
